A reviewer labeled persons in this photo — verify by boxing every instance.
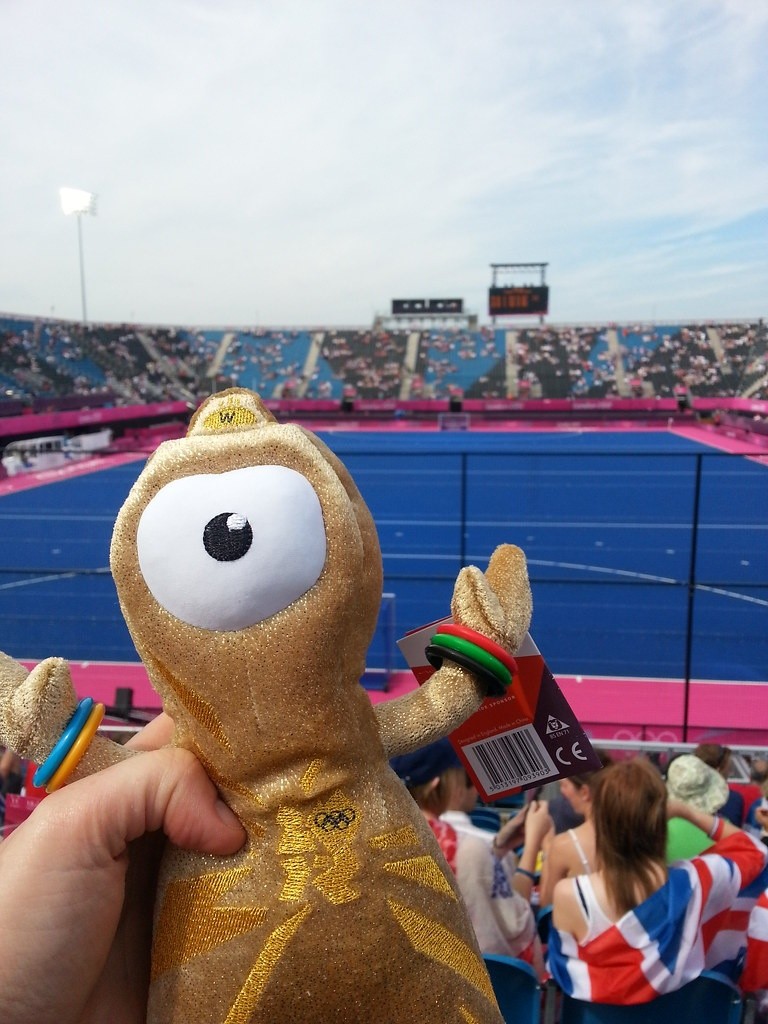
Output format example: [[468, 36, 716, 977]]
[[0, 316, 768, 412], [0, 736, 768, 1023]]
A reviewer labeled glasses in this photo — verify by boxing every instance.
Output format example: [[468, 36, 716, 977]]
[[463, 772, 473, 790]]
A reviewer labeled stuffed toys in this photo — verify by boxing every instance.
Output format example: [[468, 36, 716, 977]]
[[0, 388, 533, 1023]]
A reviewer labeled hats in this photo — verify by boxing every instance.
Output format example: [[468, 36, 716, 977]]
[[386, 734, 464, 790], [664, 755, 729, 815]]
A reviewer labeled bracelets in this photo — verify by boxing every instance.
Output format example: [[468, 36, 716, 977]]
[[707, 816, 724, 841], [516, 866, 535, 879]]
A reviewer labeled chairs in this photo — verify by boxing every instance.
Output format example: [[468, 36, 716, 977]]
[[433, 776, 752, 1024]]
